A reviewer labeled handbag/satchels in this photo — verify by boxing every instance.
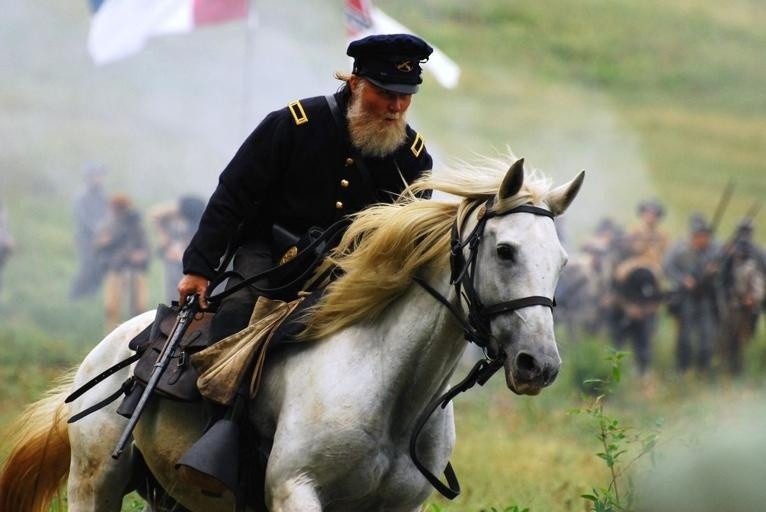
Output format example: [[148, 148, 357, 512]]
[[190, 295, 305, 405], [130, 305, 216, 402]]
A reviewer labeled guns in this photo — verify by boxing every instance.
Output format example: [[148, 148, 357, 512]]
[[112, 201, 261, 459]]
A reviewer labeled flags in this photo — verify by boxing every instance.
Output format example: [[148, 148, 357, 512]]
[[85, 0, 253, 73], [344, 0, 461, 92]]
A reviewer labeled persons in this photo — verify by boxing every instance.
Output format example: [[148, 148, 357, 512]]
[[172, 33, 437, 424], [52, 160, 207, 330], [552, 194, 766, 375]]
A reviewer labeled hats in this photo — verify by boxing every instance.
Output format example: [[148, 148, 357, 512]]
[[343, 30, 435, 95]]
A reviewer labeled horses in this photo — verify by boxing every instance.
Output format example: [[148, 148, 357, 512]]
[[0, 141, 587, 512]]
